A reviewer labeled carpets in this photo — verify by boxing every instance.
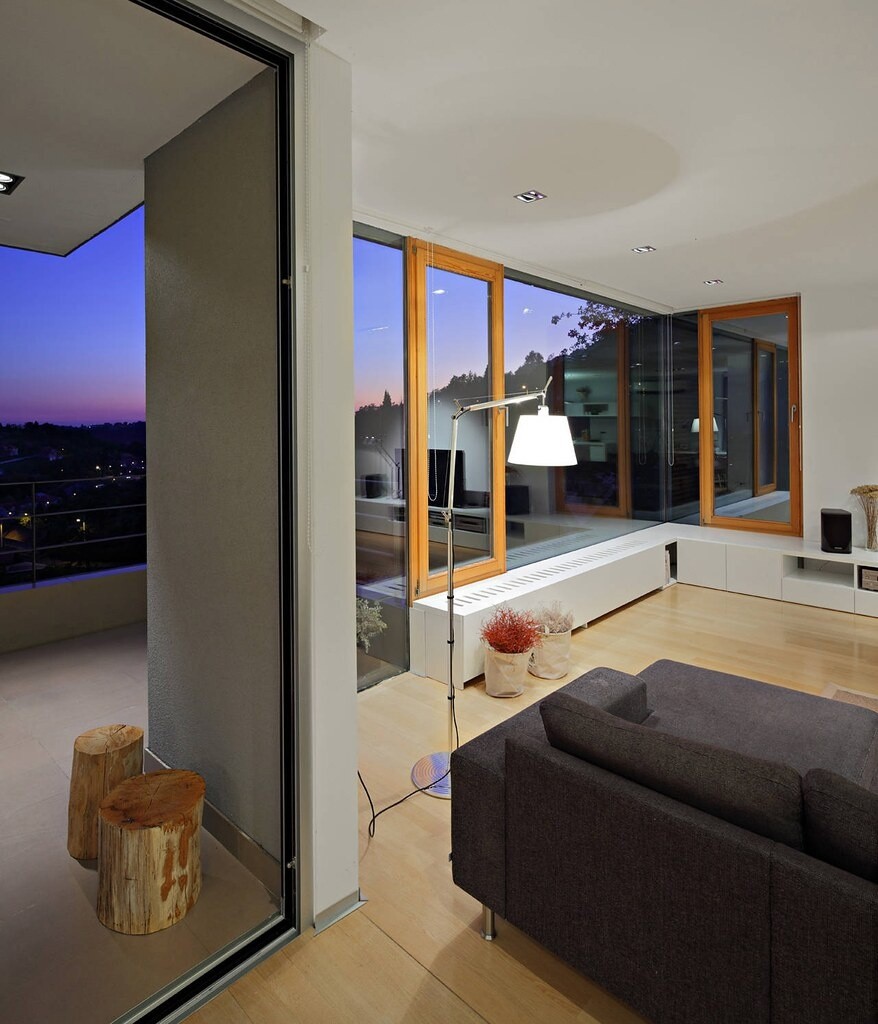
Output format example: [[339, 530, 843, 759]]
[[821, 682, 878, 713]]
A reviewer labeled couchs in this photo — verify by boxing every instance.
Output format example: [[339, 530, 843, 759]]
[[449, 658, 878, 1024]]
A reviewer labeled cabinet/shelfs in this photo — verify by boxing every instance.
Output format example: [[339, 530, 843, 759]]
[[672, 521, 878, 619], [363, 520, 655, 672], [410, 521, 676, 696], [574, 443, 610, 462], [355, 494, 628, 552]]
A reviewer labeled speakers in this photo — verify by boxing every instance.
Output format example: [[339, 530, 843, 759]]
[[820, 508, 852, 553], [366, 473, 382, 499], [505, 484, 530, 517]]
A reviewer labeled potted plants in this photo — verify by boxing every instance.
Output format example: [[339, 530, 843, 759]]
[[479, 600, 575, 699], [575, 385, 592, 402]]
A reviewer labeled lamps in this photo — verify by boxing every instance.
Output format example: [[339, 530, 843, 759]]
[[690, 418, 720, 431], [411, 377, 577, 800]]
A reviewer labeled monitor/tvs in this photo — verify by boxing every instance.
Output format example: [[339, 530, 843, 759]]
[[401, 448, 465, 509]]
